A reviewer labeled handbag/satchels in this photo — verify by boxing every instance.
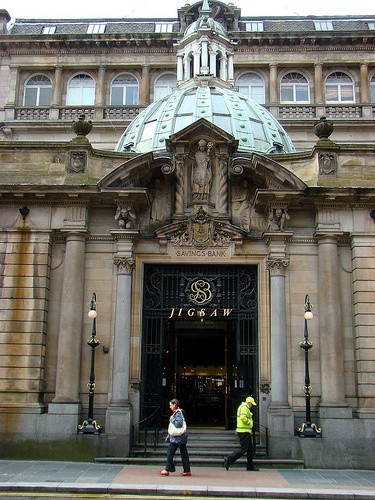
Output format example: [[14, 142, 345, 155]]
[[168, 419, 187, 437]]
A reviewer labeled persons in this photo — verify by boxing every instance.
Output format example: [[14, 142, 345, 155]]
[[267, 208, 290, 232], [157, 399, 191, 476], [115, 206, 136, 229], [223, 396, 259, 471], [192, 139, 213, 200]]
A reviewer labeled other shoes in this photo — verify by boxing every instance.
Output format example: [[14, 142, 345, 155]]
[[158, 471, 169, 476], [247, 467, 259, 471], [224, 457, 229, 471], [180, 472, 191, 476]]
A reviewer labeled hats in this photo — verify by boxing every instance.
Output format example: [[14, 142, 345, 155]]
[[246, 397, 257, 406]]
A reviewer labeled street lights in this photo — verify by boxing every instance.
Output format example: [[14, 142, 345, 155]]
[[77, 291, 104, 435], [295, 294, 322, 438]]
